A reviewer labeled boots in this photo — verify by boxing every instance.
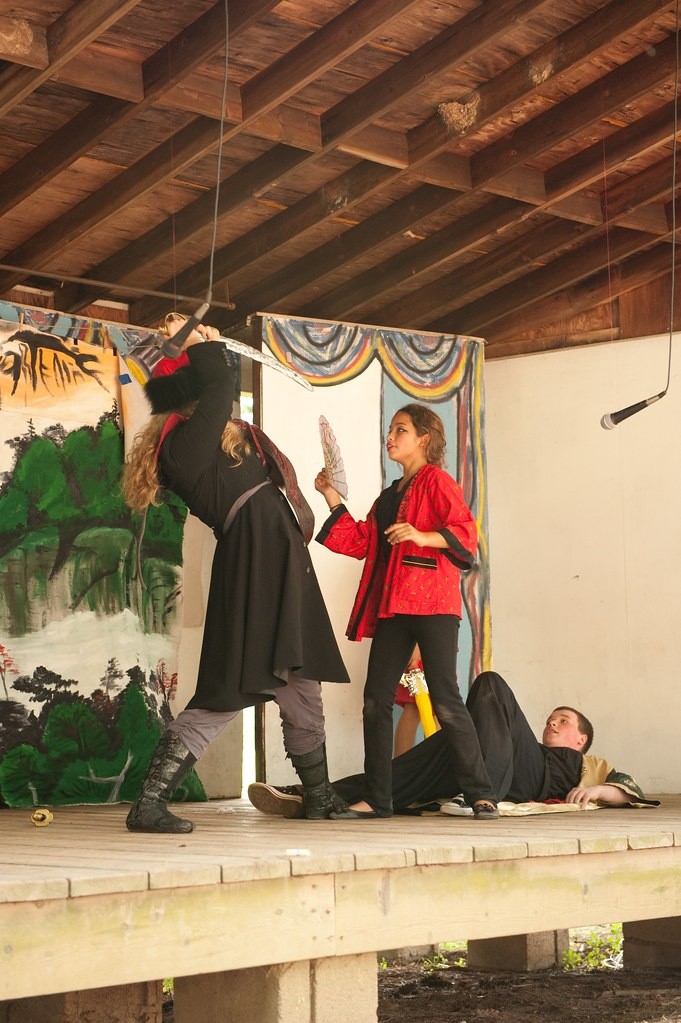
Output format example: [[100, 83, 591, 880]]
[[285, 741, 350, 821], [125, 729, 192, 833]]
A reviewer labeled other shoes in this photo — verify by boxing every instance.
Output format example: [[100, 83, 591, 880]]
[[329, 803, 379, 820], [474, 803, 499, 820]]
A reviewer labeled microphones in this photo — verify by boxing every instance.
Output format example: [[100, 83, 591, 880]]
[[601, 391, 666, 431], [161, 302, 209, 359]]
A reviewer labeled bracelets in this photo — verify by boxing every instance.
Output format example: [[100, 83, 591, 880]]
[[330, 504, 340, 511]]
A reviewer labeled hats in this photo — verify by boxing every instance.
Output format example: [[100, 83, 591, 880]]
[[143, 350, 202, 413]]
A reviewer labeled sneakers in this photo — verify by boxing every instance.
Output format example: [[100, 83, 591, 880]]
[[248, 782, 302, 815], [440, 793, 474, 816]]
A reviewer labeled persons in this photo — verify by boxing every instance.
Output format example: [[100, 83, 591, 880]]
[[393, 645, 442, 758], [314, 403, 499, 820], [248, 671, 662, 816], [127, 319, 350, 834]]
